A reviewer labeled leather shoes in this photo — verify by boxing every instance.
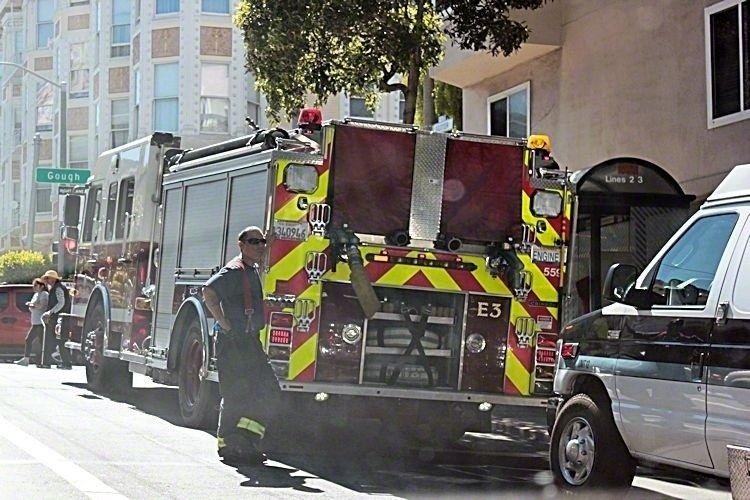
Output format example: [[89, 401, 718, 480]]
[[219, 433, 266, 464]]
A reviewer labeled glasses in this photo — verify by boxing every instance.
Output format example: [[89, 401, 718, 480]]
[[245, 238, 267, 244]]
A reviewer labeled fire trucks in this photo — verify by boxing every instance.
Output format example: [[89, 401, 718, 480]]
[[59, 107, 582, 450]]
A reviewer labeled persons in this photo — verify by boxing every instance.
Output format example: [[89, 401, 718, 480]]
[[202, 226, 283, 465], [36, 270, 72, 369], [13, 278, 49, 366]]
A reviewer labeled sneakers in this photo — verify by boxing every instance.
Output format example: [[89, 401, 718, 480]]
[[14, 357, 72, 369]]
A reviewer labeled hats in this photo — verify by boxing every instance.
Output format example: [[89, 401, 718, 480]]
[[32, 270, 62, 287]]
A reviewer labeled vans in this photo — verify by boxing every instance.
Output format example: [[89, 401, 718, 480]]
[[549, 163, 750, 500]]
